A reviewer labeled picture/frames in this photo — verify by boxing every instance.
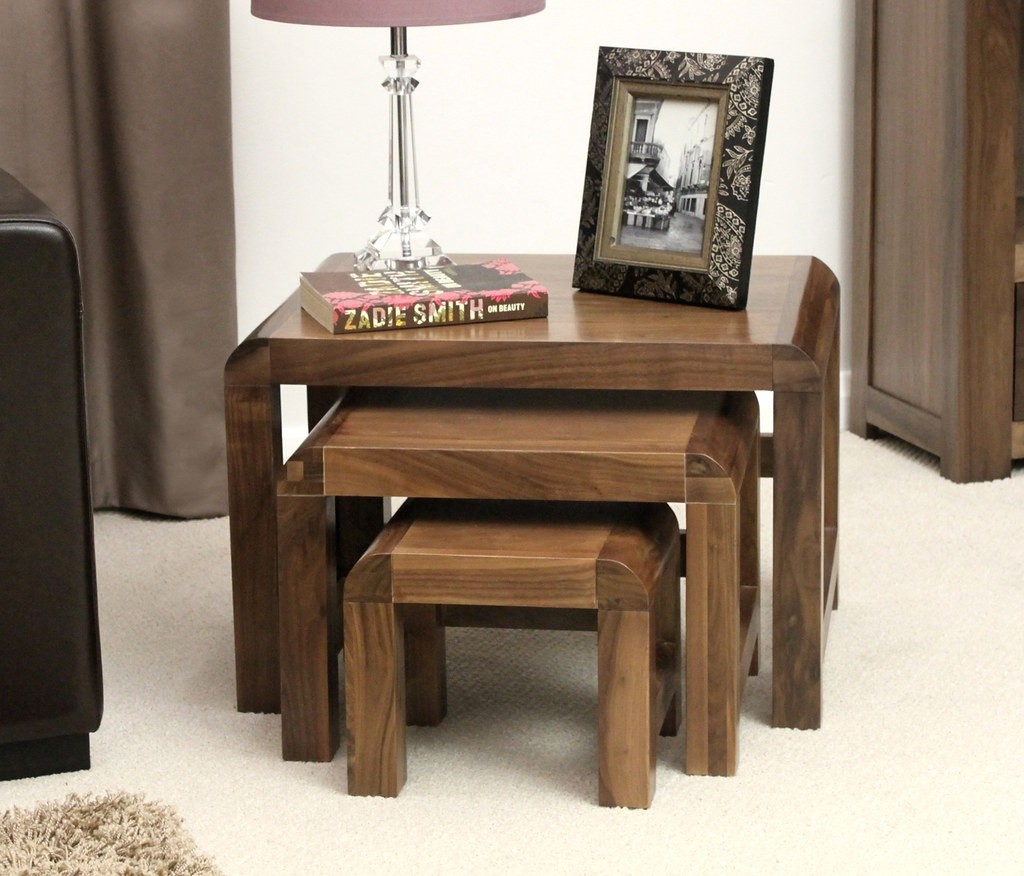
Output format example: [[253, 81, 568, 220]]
[[571, 48, 773, 312]]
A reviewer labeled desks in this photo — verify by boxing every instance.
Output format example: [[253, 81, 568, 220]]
[[223, 255, 840, 729]]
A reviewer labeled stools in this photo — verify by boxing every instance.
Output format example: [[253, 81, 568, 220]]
[[273, 390, 761, 778], [346, 496, 684, 806]]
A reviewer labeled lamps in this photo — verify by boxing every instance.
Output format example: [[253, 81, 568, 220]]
[[249, 1, 550, 270]]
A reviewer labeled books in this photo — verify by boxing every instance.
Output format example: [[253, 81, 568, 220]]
[[300, 258, 548, 335]]
